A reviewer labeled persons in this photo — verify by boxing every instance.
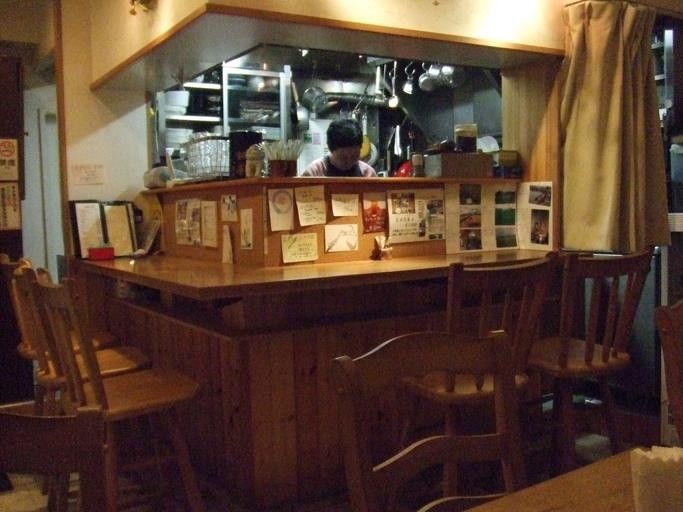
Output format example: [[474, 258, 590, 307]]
[[297, 119, 379, 176]]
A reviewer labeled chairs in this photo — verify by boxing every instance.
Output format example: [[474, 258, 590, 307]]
[[534, 247, 657, 473], [330, 328, 519, 511], [655, 295, 683, 441], [389, 250, 557, 493]]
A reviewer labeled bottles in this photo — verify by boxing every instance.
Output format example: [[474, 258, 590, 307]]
[[411, 153, 423, 177]]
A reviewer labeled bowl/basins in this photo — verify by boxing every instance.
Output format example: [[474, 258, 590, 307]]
[[165, 89, 194, 159], [86, 247, 116, 261], [269, 158, 296, 178]]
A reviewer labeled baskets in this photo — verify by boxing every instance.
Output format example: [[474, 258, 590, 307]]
[[184, 135, 231, 178]]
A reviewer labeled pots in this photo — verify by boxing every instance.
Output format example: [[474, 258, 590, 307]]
[[416, 66, 480, 94], [291, 66, 326, 131]]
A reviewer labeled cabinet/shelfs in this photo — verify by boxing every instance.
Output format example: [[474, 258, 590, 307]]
[[155, 61, 293, 177]]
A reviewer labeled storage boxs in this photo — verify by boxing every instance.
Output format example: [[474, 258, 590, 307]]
[[421, 152, 494, 181]]
